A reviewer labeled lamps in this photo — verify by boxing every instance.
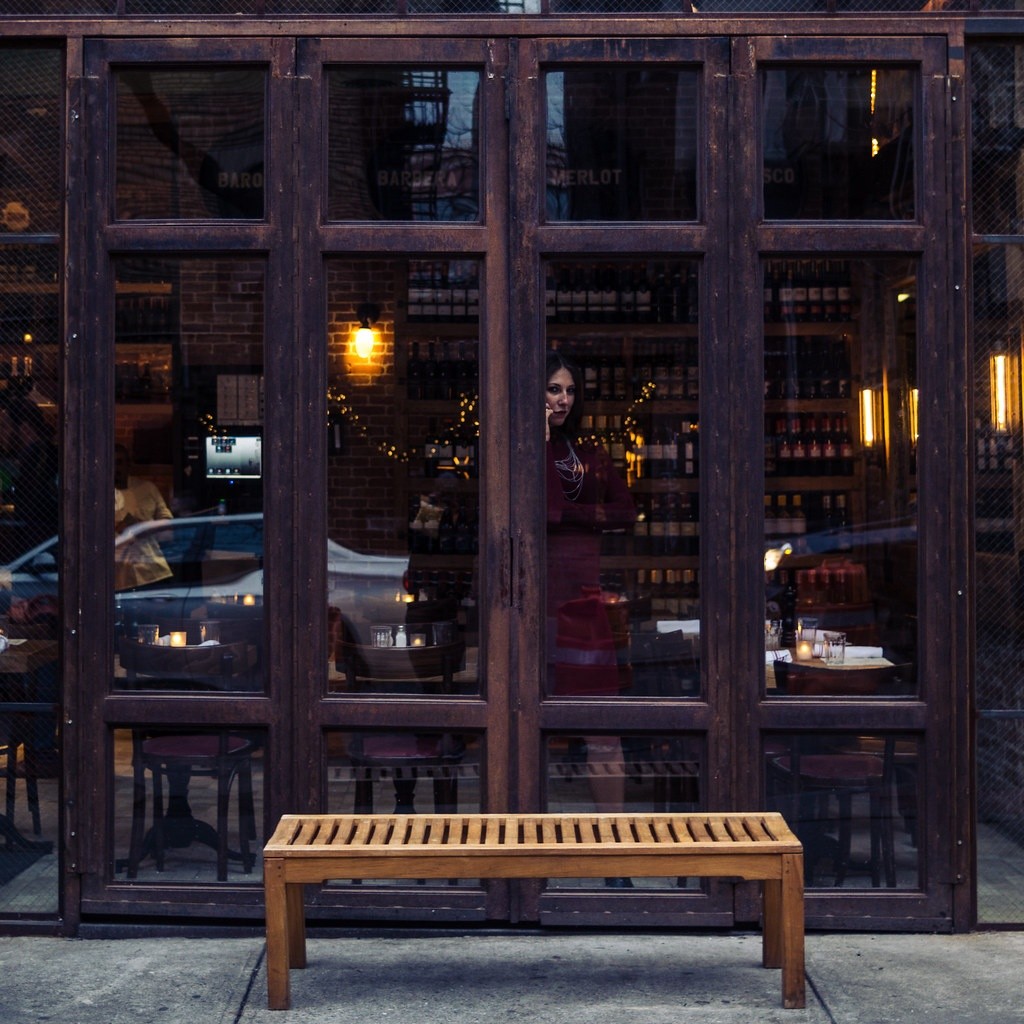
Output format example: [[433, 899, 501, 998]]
[[909, 383, 917, 449], [857, 382, 877, 454], [355, 300, 382, 359], [988, 338, 1013, 440]]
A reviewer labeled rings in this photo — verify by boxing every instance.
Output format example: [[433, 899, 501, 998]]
[[546, 406, 550, 409]]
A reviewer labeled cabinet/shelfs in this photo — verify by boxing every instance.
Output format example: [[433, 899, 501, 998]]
[[391, 256, 870, 603], [0, 282, 173, 415]]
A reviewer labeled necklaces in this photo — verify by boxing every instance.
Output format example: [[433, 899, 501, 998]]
[[555, 433, 585, 501]]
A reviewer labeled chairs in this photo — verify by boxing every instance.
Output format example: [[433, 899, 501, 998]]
[[630, 655, 702, 812], [768, 659, 915, 888], [334, 639, 466, 887], [815, 733, 917, 848], [119, 637, 257, 881]]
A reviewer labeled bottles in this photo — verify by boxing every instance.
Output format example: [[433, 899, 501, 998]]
[[406, 251, 874, 649], [395, 626, 406, 647]]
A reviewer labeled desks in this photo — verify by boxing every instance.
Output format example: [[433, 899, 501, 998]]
[[326, 647, 478, 813], [0, 638, 60, 857], [113, 653, 258, 874], [766, 647, 894, 874]]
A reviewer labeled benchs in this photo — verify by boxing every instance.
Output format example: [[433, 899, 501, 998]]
[[263, 814, 807, 1009]]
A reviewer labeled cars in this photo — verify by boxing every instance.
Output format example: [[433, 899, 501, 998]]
[[0, 514, 407, 680]]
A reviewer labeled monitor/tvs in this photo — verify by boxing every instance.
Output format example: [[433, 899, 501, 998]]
[[194, 424, 264, 487]]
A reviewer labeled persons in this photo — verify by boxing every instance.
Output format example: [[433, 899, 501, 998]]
[[546, 351, 636, 811], [0, 399, 57, 521], [113, 444, 176, 593]]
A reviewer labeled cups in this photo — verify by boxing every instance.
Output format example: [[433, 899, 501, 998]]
[[137, 623, 159, 645], [199, 621, 220, 644], [824, 631, 846, 665], [370, 626, 393, 647]]
